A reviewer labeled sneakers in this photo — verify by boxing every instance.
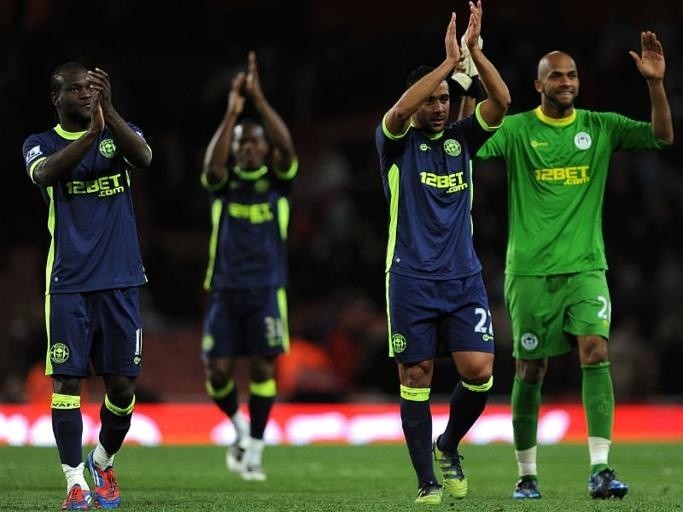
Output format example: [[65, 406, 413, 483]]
[[432, 434, 468, 498], [62, 484, 93, 511], [514, 475, 540, 499], [85, 449, 120, 508], [588, 469, 629, 499], [415, 485, 443, 505], [226, 428, 266, 480]]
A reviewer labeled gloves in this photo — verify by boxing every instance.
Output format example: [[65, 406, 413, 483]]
[[453, 32, 487, 99]]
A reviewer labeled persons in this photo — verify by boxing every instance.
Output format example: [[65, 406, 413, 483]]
[[272, 175, 401, 404], [22, 62, 152, 511], [200, 50, 300, 483], [372, 0, 513, 506], [0, 175, 216, 405], [452, 0, 673, 501], [470, 175, 682, 404]]
